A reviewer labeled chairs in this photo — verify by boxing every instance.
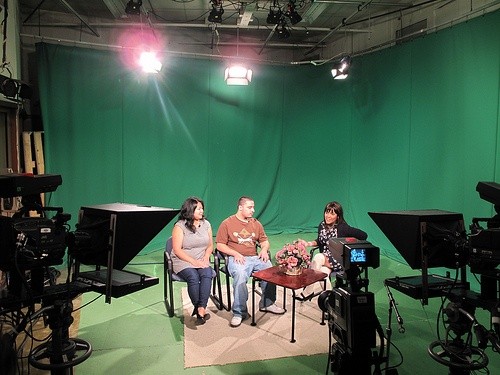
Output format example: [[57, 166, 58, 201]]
[[163, 236, 222, 318], [213, 243, 271, 312]]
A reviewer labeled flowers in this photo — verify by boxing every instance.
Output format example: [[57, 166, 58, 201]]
[[275, 239, 312, 268]]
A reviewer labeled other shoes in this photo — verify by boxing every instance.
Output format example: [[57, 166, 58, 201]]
[[293, 291, 314, 302], [259, 303, 286, 314], [230, 316, 243, 327]]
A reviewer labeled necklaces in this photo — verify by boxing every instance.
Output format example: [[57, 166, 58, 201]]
[[235, 215, 249, 224]]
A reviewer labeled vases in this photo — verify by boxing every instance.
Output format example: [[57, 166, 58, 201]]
[[283, 266, 304, 275]]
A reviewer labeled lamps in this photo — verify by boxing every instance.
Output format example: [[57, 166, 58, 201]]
[[224, 65, 253, 86], [125, 0, 142, 15], [266, 0, 303, 39], [208, 0, 224, 23], [315, 52, 353, 80]]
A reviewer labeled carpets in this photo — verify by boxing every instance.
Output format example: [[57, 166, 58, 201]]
[[182, 283, 387, 369]]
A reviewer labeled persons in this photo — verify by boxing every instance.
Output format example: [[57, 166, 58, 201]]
[[216, 196, 285, 327], [295, 202, 368, 321], [169, 197, 214, 324]]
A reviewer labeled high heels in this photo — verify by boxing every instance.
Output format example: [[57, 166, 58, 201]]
[[191, 306, 211, 324]]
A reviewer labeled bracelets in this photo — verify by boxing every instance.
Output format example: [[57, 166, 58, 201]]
[[308, 241, 313, 247]]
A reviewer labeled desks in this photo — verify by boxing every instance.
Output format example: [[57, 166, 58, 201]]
[[252, 266, 328, 343]]
